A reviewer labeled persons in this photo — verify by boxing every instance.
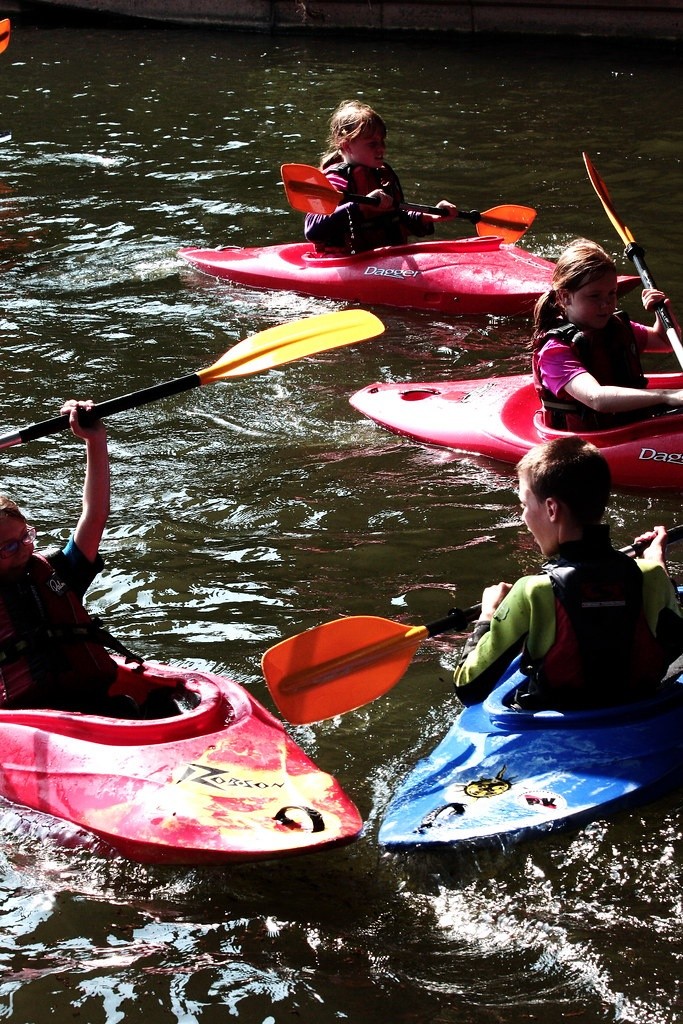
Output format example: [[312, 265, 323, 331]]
[[454, 436, 683, 710], [304, 99, 458, 255], [0, 399, 145, 719], [532, 238, 683, 432]]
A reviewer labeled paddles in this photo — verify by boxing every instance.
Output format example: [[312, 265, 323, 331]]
[[281, 164, 538, 242], [261, 521, 683, 726], [0, 308, 386, 449], [582, 152, 683, 378]]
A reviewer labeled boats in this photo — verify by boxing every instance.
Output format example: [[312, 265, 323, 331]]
[[0, 654, 366, 868], [349, 369, 683, 490], [377, 584, 682, 852], [177, 235, 645, 319]]
